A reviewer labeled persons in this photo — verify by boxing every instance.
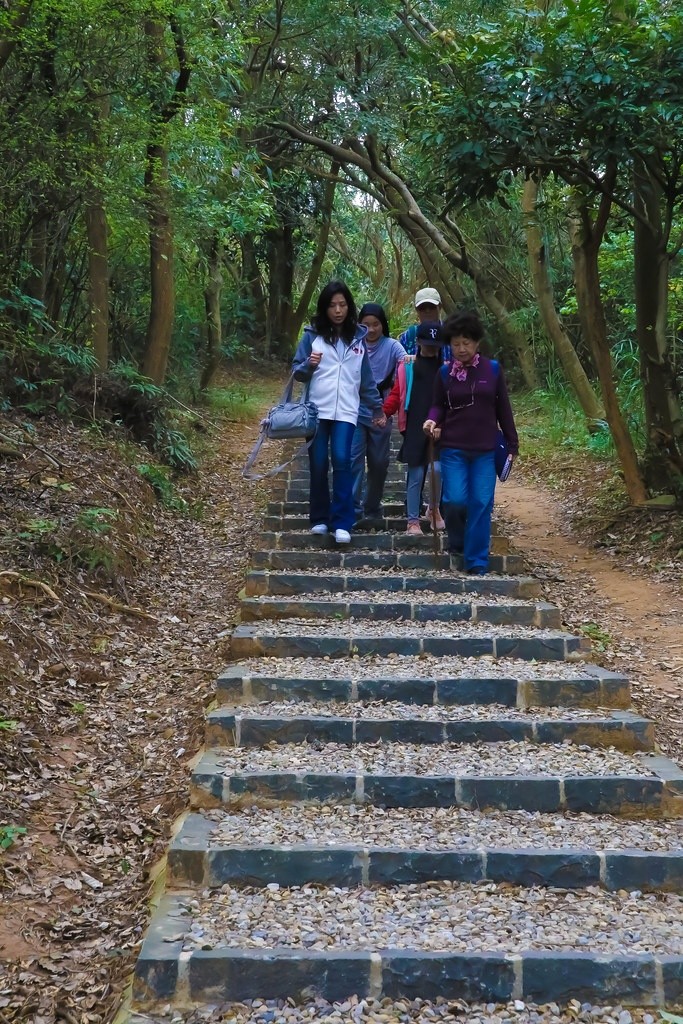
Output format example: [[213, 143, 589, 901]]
[[290, 281, 388, 543], [371, 321, 449, 536], [422, 313, 519, 576], [356, 304, 417, 532], [392, 287, 452, 520]]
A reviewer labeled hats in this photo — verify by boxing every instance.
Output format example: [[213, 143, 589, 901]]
[[418, 319, 446, 348], [413, 287, 443, 308]]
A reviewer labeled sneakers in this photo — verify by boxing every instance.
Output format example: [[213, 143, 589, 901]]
[[405, 504, 446, 536], [312, 524, 352, 545]]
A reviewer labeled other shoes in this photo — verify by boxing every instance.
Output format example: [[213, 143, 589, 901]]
[[353, 507, 384, 529], [444, 545, 486, 577]]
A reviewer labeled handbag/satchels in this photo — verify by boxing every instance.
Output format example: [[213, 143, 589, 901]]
[[267, 401, 318, 438]]
